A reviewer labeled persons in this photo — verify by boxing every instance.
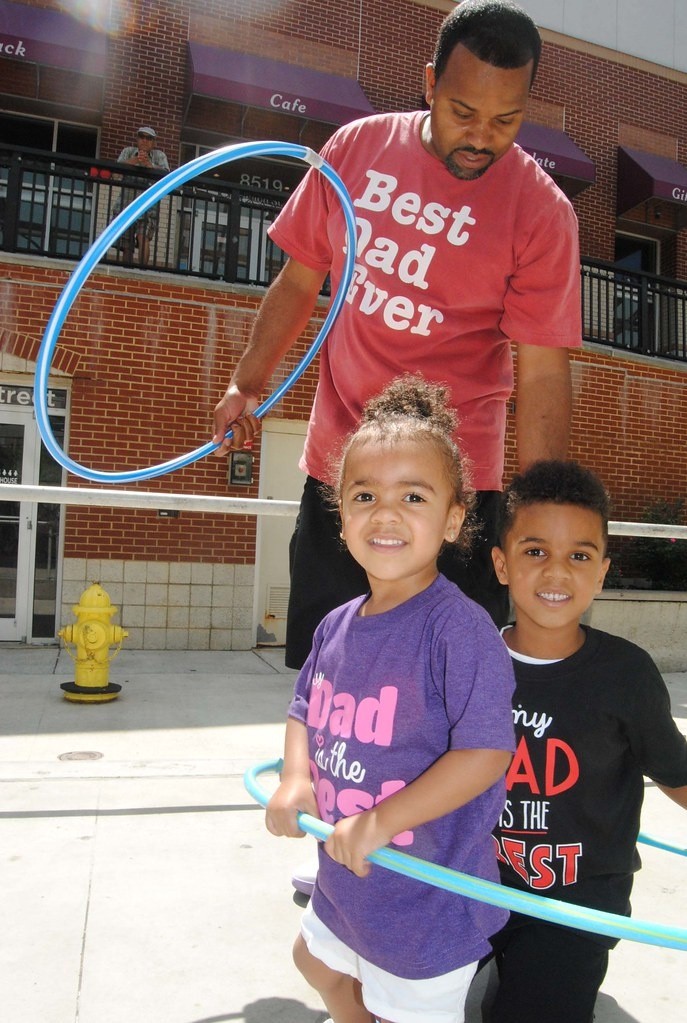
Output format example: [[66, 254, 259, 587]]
[[211, 0, 583, 897], [110, 126, 170, 265], [474, 459, 687, 1022], [264, 374, 511, 1023]]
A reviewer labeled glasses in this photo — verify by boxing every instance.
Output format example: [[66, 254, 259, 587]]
[[138, 133, 155, 140]]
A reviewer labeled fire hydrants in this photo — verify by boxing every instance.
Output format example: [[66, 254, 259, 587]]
[[55, 579, 129, 704]]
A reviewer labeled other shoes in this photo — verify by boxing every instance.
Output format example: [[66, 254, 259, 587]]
[[292, 856, 319, 895]]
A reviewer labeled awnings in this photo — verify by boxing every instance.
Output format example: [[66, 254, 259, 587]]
[[617, 146, 687, 217], [0, 0, 108, 75], [184, 42, 377, 128], [512, 122, 596, 184]]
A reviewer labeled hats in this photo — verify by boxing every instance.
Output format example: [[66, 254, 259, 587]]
[[137, 126, 156, 137]]
